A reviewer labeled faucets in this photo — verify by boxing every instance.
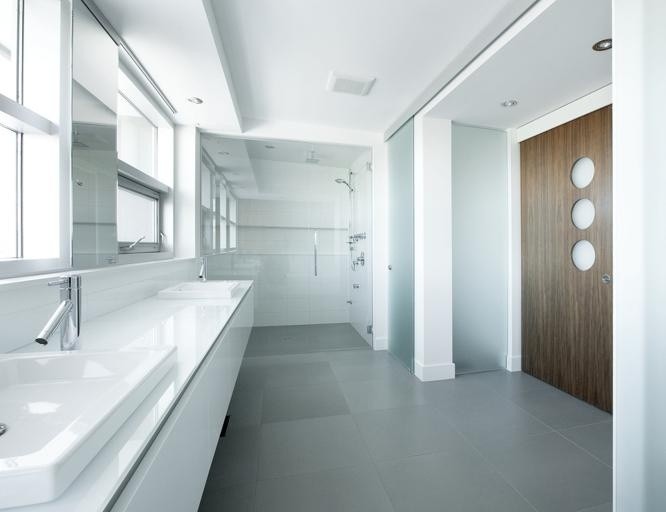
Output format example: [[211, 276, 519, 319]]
[[33, 272, 84, 352], [197, 254, 208, 282]]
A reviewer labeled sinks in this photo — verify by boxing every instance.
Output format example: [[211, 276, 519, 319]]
[[0, 336, 179, 511], [157, 280, 242, 299]]
